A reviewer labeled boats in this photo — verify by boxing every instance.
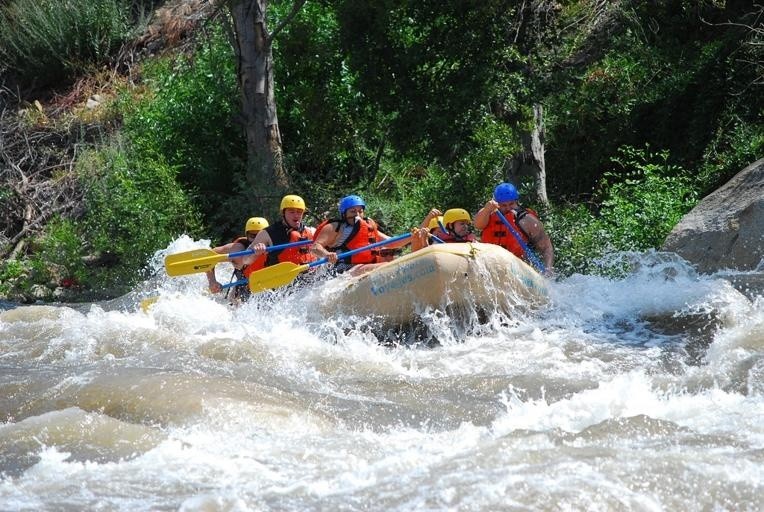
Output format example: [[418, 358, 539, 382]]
[[235, 240, 559, 338]]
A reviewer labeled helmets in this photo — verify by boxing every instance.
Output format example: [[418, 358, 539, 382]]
[[494, 182, 520, 203], [442, 207, 472, 228], [428, 216, 444, 232], [244, 216, 269, 234], [279, 194, 306, 213], [339, 195, 366, 214]]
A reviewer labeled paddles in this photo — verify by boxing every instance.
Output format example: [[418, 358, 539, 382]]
[[139, 279, 250, 316], [250, 233, 411, 294], [164, 239, 315, 277]]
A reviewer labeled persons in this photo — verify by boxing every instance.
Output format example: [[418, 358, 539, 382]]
[[205, 217, 270, 299], [312, 196, 421, 279], [243, 195, 320, 283], [419, 183, 555, 278]]
